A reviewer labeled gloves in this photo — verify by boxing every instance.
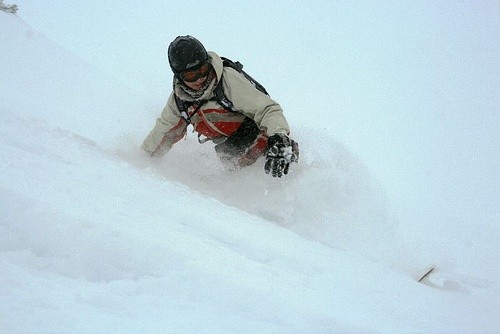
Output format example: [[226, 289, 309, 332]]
[[263, 133, 291, 178]]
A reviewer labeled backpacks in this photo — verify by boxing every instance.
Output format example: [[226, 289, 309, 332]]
[[174, 56, 270, 113]]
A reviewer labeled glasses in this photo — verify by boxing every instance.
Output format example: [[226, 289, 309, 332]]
[[177, 58, 210, 82]]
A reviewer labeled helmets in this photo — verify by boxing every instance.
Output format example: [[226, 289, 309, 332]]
[[168, 35, 210, 72]]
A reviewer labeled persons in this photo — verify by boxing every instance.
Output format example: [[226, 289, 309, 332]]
[[140, 34, 299, 179]]
